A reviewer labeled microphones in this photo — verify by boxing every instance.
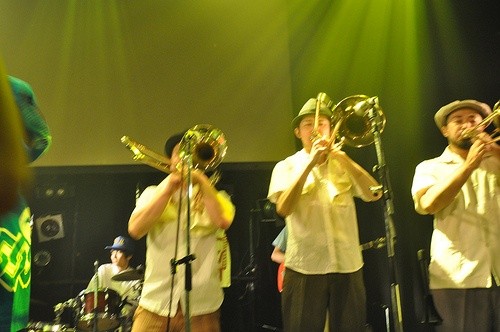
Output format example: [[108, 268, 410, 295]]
[[353, 96, 375, 117]]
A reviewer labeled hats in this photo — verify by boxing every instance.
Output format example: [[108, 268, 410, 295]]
[[291, 97, 334, 129], [434, 99, 492, 130], [104, 235, 134, 250], [165, 128, 190, 157]]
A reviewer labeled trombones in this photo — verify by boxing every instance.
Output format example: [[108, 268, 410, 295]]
[[122, 121, 227, 207], [311, 91, 388, 160], [461, 98, 500, 154]]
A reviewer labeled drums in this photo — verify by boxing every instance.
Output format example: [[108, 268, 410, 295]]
[[19, 287, 121, 332]]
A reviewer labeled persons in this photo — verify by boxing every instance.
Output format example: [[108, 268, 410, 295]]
[[266, 97, 382, 332], [0, 61, 52, 332], [411, 100, 500, 332], [271, 225, 289, 292], [128, 134, 236, 332], [79, 235, 142, 332]]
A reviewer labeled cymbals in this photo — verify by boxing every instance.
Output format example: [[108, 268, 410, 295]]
[[111, 266, 145, 282]]
